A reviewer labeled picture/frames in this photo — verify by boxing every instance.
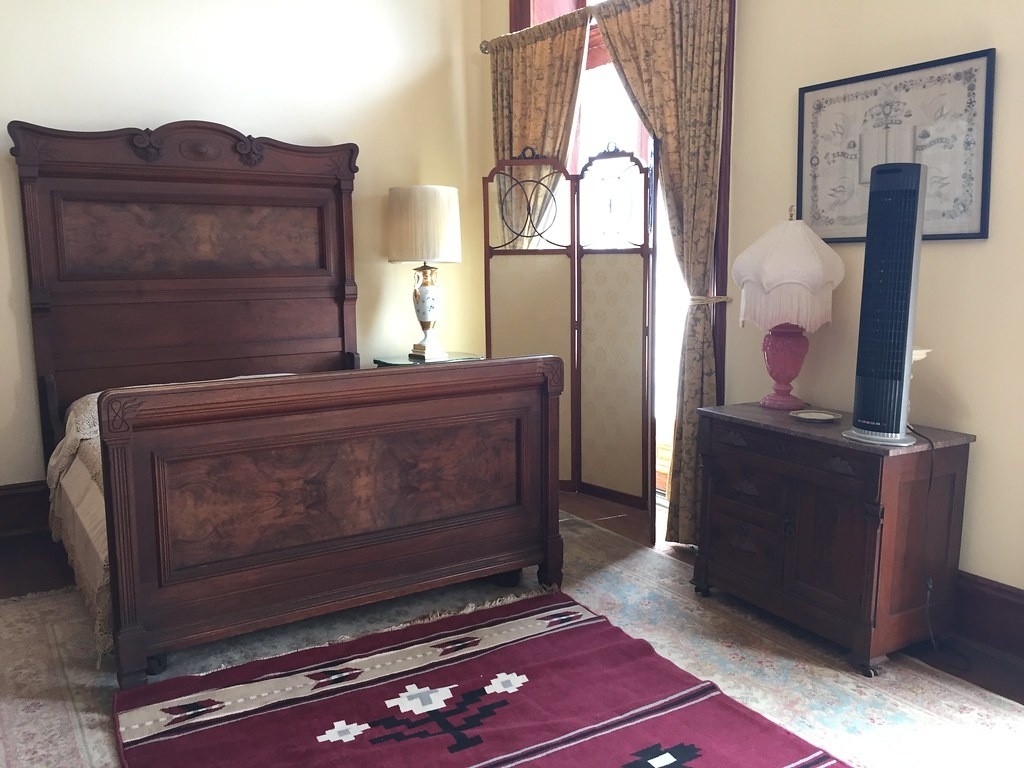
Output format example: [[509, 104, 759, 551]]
[[797, 48, 996, 243]]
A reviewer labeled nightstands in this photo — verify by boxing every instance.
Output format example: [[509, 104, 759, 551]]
[[374, 352, 484, 368]]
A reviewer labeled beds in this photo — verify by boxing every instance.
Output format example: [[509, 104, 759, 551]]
[[8, 120, 563, 690]]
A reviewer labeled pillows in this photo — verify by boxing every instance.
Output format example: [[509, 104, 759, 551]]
[[64, 373, 297, 440]]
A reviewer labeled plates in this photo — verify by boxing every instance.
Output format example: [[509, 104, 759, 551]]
[[789, 410, 842, 423]]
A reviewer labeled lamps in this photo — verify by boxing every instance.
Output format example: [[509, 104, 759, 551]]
[[387, 185, 463, 362], [732, 205, 845, 409]]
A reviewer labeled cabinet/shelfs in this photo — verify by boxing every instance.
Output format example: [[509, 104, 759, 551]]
[[690, 402, 977, 677]]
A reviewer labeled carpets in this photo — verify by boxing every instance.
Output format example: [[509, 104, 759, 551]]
[[113, 583, 853, 768], [0, 510, 1024, 768]]
